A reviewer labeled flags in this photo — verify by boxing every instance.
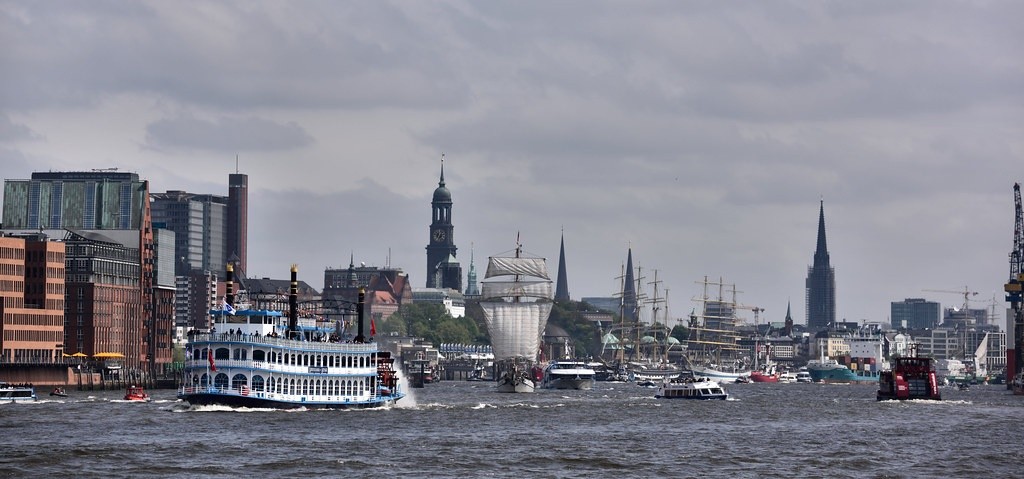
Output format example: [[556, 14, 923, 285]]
[[208, 351, 216, 373], [223, 300, 236, 316]]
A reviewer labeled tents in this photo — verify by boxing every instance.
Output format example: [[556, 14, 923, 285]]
[[72, 352, 88, 358], [93, 351, 126, 358], [63, 352, 71, 357]]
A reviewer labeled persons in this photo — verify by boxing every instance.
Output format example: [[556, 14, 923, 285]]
[[237, 327, 242, 341], [313, 332, 327, 343], [3, 382, 35, 389], [254, 330, 258, 336], [290, 333, 306, 341], [271, 332, 277, 337], [266, 332, 270, 337], [346, 339, 365, 344], [329, 333, 340, 342], [670, 377, 710, 383], [186, 328, 200, 337], [230, 328, 234, 336], [53, 386, 66, 395]]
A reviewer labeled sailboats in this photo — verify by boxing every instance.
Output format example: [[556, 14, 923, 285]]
[[593, 258, 682, 383], [474, 229, 557, 395], [678, 274, 761, 384]]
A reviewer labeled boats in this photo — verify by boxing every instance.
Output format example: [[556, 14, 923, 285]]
[[750, 362, 811, 384], [124, 386, 150, 403], [541, 353, 596, 390], [934, 358, 999, 386], [652, 377, 732, 401], [0, 387, 38, 406], [407, 352, 440, 382], [807, 337, 881, 385], [176, 261, 405, 408], [50, 388, 68, 397], [873, 341, 941, 401], [467, 360, 490, 381]]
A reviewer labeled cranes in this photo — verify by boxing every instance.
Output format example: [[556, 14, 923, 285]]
[[735, 305, 764, 332], [922, 286, 979, 352]]
